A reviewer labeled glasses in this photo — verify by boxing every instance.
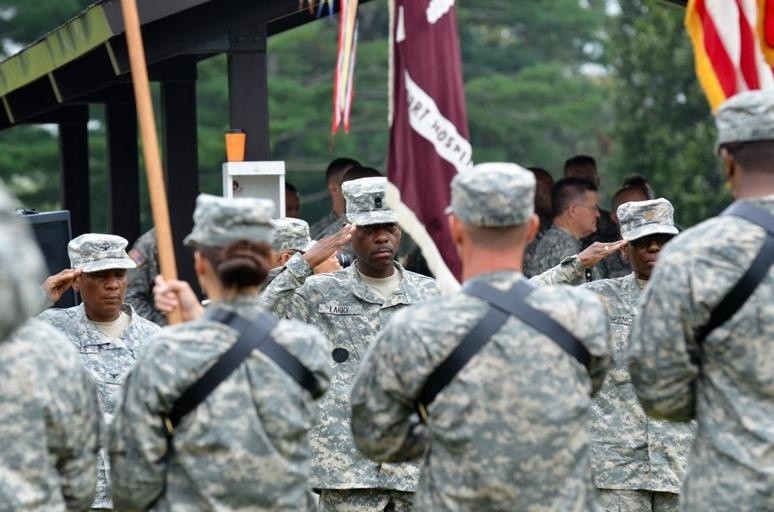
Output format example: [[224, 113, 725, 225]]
[[566, 204, 599, 212]]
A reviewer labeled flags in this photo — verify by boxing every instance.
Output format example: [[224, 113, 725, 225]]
[[296, 1, 361, 133], [387, 1, 495, 286], [683, 1, 770, 116]]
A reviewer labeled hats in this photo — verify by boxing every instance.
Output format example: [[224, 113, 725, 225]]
[[615, 198, 679, 241], [66, 231, 137, 273], [184, 190, 280, 246], [277, 218, 318, 251], [715, 89, 774, 151], [340, 176, 398, 227], [444, 163, 536, 229]]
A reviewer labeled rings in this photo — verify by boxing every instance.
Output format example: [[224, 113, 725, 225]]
[[605, 245, 609, 251]]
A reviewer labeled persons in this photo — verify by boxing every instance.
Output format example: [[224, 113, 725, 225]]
[[1, 317, 107, 511], [611, 177, 655, 240], [522, 165, 555, 278], [124, 227, 168, 327], [310, 157, 362, 240], [315, 168, 380, 264], [350, 161, 611, 510], [532, 197, 696, 512], [269, 219, 319, 268], [564, 156, 622, 248], [255, 176, 441, 511], [533, 179, 601, 285], [286, 182, 303, 219], [110, 192, 334, 512], [40, 234, 163, 511], [626, 91, 770, 512]]
